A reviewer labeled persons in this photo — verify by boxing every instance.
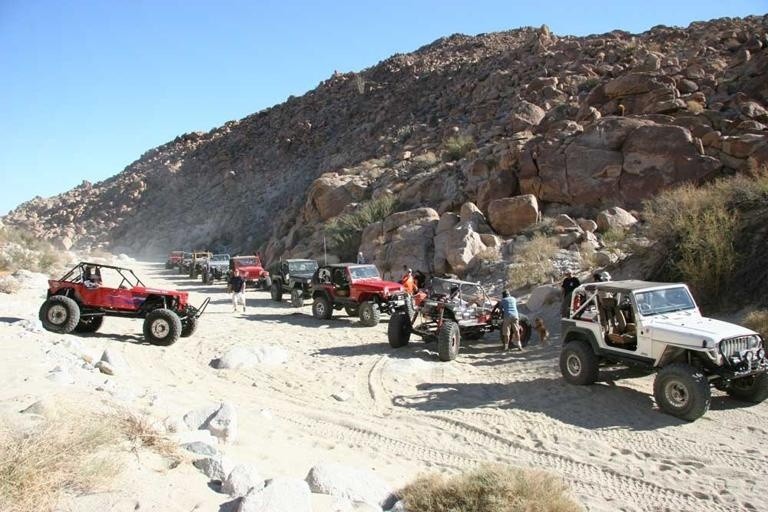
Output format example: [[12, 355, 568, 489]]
[[400, 264, 408, 277], [402, 268, 418, 295], [491, 289, 523, 352], [558, 269, 580, 318], [227, 269, 246, 313], [415, 270, 425, 292]]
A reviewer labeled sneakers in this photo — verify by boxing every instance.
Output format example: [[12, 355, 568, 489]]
[[519, 346, 523, 351], [504, 349, 510, 352]]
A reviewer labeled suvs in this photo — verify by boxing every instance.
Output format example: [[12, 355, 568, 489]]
[[39, 262, 210, 347], [560, 280, 768, 421]]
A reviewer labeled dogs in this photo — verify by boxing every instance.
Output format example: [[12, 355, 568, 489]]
[[532, 317, 549, 345]]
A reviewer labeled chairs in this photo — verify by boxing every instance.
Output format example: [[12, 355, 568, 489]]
[[599, 298, 653, 348]]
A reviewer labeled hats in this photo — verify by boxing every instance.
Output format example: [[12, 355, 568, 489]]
[[600, 272, 611, 280], [565, 269, 572, 274], [503, 290, 510, 297]]
